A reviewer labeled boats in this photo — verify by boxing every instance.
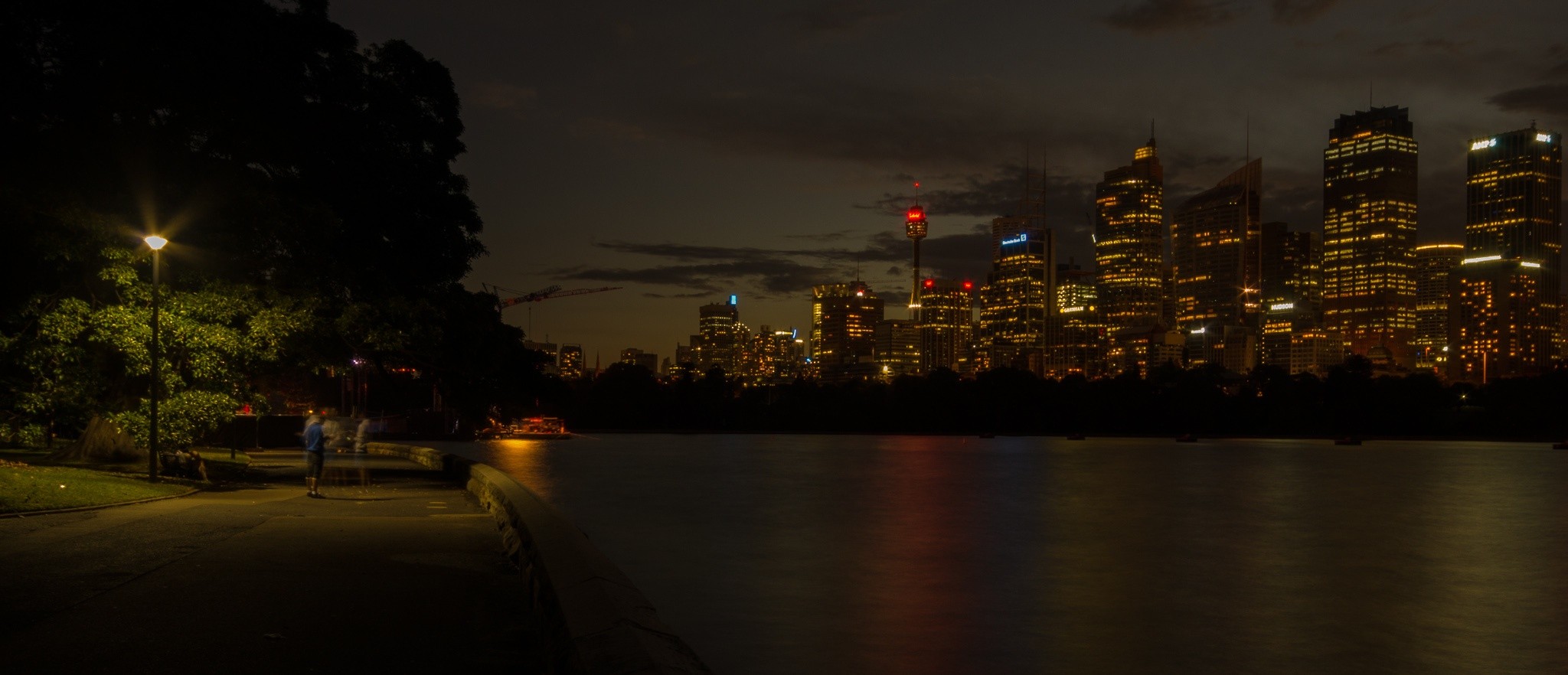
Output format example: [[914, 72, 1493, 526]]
[[512, 417, 572, 439]]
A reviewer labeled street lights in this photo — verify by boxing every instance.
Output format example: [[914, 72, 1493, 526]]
[[145, 236, 169, 480]]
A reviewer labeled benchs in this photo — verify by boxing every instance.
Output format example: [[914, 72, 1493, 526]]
[[158, 447, 182, 476], [207, 461, 248, 482]]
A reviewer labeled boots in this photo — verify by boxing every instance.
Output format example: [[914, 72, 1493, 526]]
[[305, 477, 311, 496], [311, 478, 322, 498]]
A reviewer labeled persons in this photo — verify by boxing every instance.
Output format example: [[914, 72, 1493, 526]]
[[302, 407, 384, 499]]
